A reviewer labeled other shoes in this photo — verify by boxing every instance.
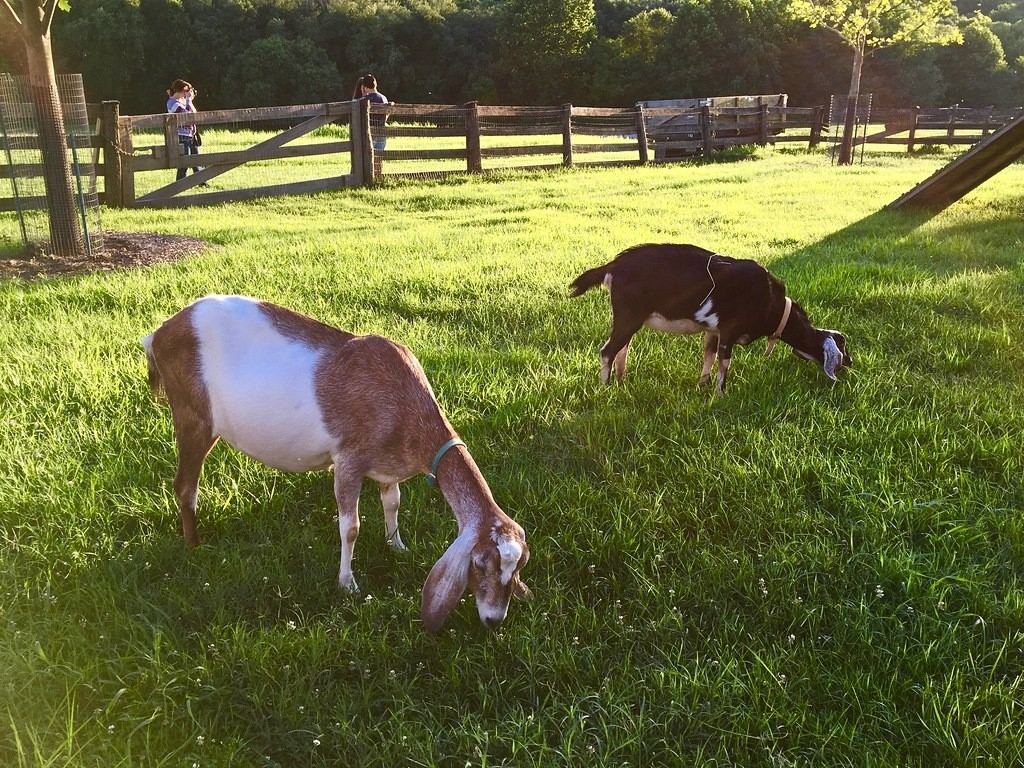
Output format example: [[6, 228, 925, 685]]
[[199, 183, 210, 188]]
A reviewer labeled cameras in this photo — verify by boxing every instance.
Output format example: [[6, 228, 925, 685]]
[[187, 89, 197, 96]]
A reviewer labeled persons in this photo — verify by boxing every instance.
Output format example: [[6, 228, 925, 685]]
[[353, 75, 388, 179], [167, 79, 210, 188]]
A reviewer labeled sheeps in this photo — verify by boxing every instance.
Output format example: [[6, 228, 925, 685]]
[[566, 244, 851, 399], [137, 296, 535, 637]]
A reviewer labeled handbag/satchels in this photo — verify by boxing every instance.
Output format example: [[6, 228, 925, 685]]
[[193, 130, 202, 146]]
